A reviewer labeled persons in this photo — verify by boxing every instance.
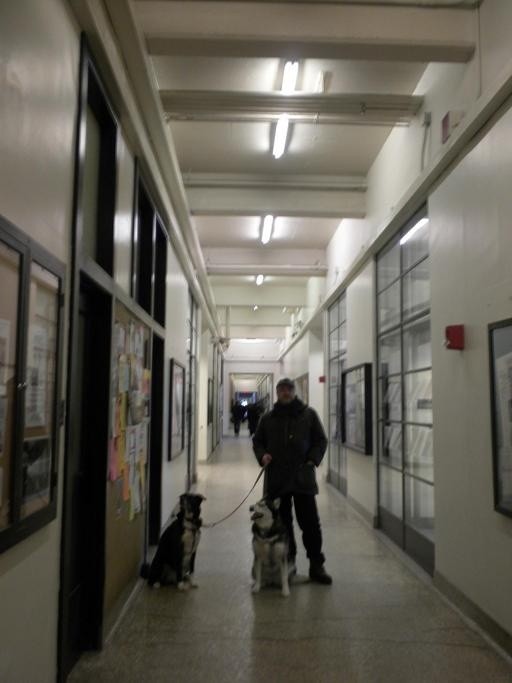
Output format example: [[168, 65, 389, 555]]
[[230, 397, 266, 440], [253, 379, 333, 585]]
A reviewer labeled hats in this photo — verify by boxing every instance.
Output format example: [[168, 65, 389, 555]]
[[277, 379, 295, 387]]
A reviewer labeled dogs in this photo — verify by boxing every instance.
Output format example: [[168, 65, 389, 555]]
[[248, 494, 298, 598], [137, 493, 208, 591]]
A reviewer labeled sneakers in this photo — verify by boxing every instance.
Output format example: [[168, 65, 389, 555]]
[[309, 571, 332, 584]]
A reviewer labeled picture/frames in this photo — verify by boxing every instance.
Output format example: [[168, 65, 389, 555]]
[[169, 357, 186, 460], [339, 362, 371, 456]]
